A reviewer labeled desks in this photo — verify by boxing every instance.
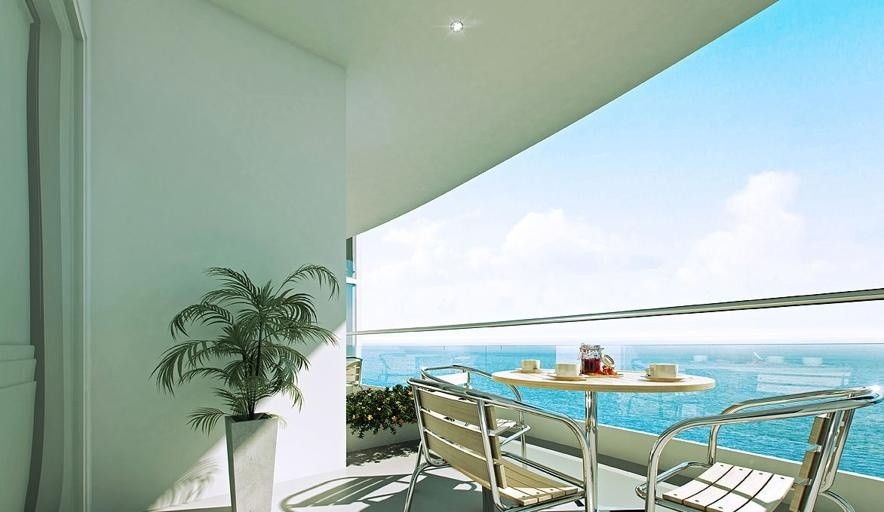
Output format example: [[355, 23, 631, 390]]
[[490, 368, 715, 512]]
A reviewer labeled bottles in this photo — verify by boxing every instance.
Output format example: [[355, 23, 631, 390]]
[[579, 343, 615, 375]]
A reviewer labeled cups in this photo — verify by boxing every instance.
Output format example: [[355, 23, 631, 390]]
[[556, 363, 576, 377], [645, 363, 679, 378], [522, 359, 541, 370]]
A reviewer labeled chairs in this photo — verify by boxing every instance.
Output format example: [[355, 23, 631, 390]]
[[636, 386, 883, 512], [403, 377, 599, 512], [414, 364, 532, 461]]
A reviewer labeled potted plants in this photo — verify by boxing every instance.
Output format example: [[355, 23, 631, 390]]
[[147, 262, 342, 512]]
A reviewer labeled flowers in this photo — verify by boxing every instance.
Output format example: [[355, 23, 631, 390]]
[[346, 384, 417, 440]]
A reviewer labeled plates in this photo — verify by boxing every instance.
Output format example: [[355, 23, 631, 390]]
[[547, 374, 583, 380], [646, 375, 685, 381], [517, 368, 543, 374]]
